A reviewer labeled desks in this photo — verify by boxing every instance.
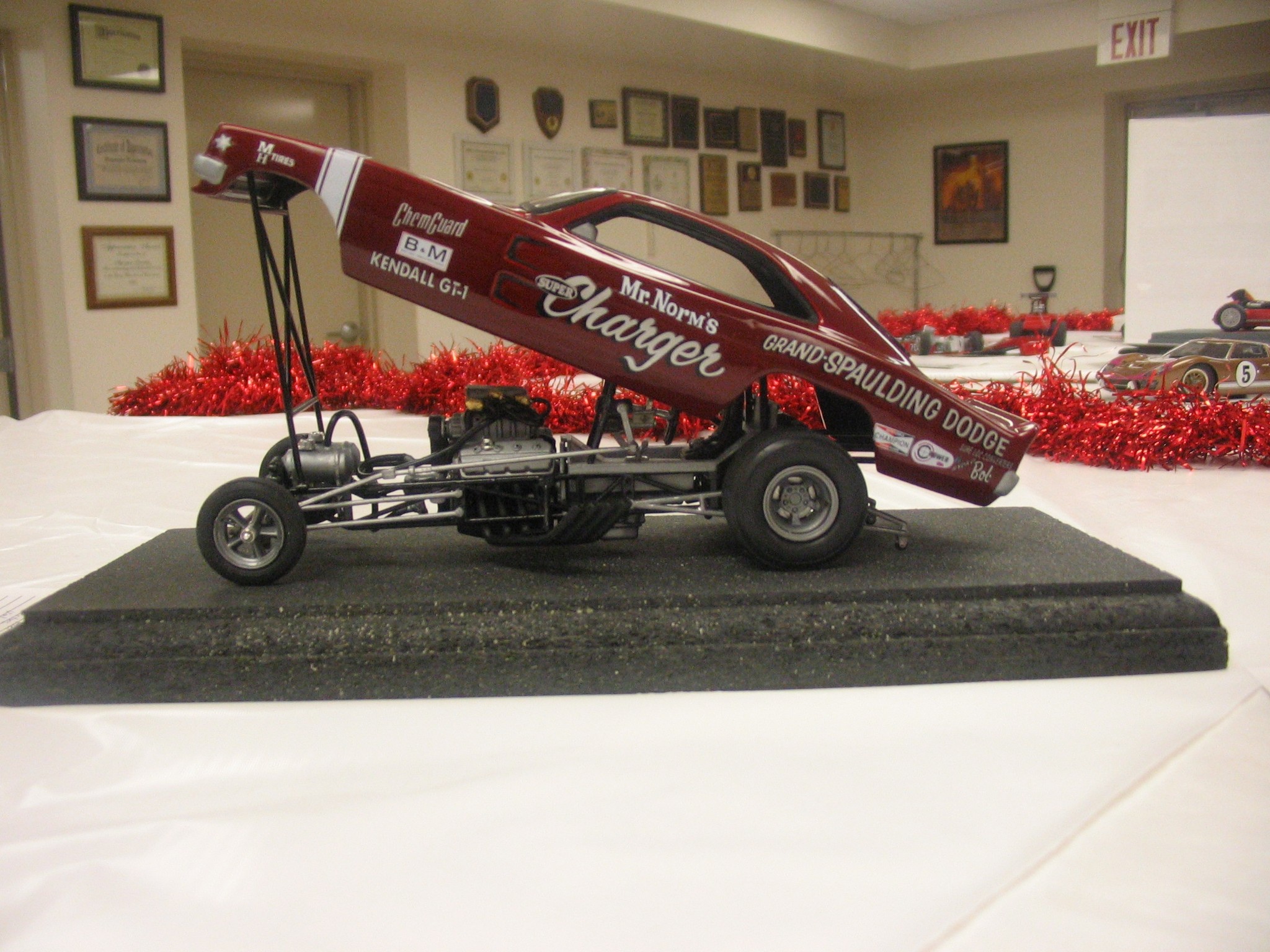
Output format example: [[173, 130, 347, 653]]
[[0, 409, 1270, 951]]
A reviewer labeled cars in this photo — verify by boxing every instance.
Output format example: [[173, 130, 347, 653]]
[[1211, 289, 1270, 332], [1095, 337, 1270, 402], [186, 120, 1043, 587]]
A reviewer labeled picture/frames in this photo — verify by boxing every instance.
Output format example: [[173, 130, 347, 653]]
[[933, 140, 1008, 244]]
